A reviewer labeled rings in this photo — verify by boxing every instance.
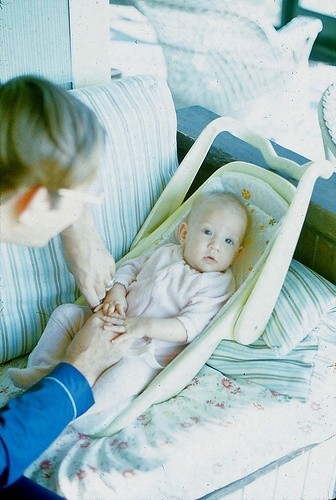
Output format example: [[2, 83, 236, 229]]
[[104, 279, 115, 288]]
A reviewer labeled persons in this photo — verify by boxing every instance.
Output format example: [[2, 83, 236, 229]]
[[6, 190, 249, 438], [0, 74, 125, 491]]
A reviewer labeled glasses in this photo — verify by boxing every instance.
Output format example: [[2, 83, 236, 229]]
[[47, 184, 105, 207]]
